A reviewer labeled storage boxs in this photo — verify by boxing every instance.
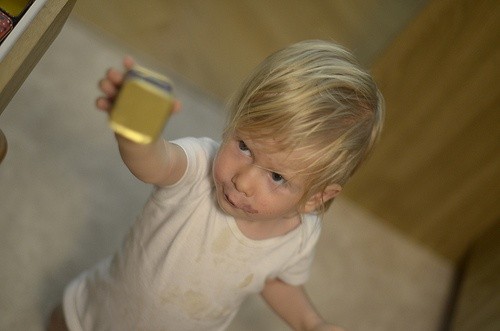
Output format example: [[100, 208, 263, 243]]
[[108, 66, 177, 147]]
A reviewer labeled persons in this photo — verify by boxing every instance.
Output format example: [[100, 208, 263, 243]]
[[47, 39, 384, 331]]
